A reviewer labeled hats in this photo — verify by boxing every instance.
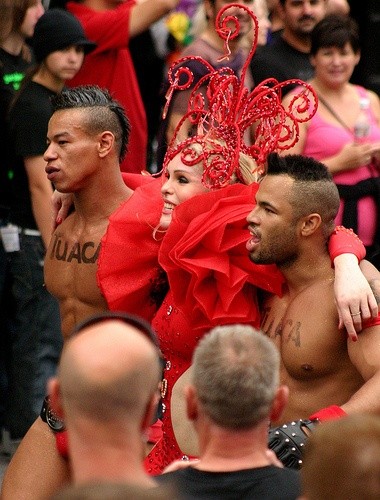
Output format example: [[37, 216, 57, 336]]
[[33, 9, 97, 64]]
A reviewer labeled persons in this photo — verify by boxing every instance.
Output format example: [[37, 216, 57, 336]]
[[0, 0, 380, 500]]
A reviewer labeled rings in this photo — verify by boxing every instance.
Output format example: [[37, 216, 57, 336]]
[[351, 312, 361, 316]]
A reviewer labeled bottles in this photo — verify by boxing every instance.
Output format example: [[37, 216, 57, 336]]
[[355, 99, 371, 143]]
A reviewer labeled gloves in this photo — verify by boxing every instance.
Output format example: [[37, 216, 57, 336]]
[[267, 418, 321, 468]]
